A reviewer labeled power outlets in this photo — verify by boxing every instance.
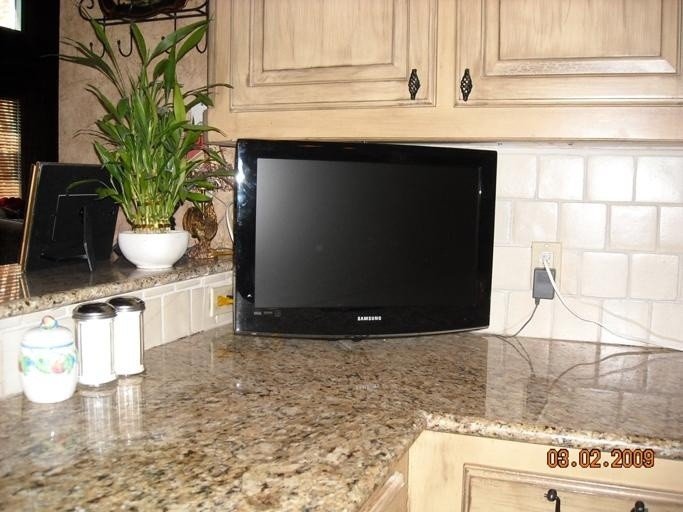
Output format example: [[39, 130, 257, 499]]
[[530, 240, 563, 299], [209, 284, 235, 318]]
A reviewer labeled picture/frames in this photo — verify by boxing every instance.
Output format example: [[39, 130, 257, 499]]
[[19, 160, 120, 272]]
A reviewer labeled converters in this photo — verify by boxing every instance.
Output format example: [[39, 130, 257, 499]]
[[533, 268, 556, 299]]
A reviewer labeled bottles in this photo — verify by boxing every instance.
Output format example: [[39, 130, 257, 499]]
[[17, 315, 78, 403], [72, 296, 147, 389]]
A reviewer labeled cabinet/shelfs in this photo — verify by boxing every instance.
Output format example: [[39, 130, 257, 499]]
[[205, 0, 683, 143], [409, 431, 682, 511]]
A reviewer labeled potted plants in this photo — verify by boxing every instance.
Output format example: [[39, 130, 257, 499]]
[[47, 7, 237, 276]]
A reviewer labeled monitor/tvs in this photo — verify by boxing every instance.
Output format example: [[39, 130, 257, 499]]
[[232, 138, 497, 340]]
